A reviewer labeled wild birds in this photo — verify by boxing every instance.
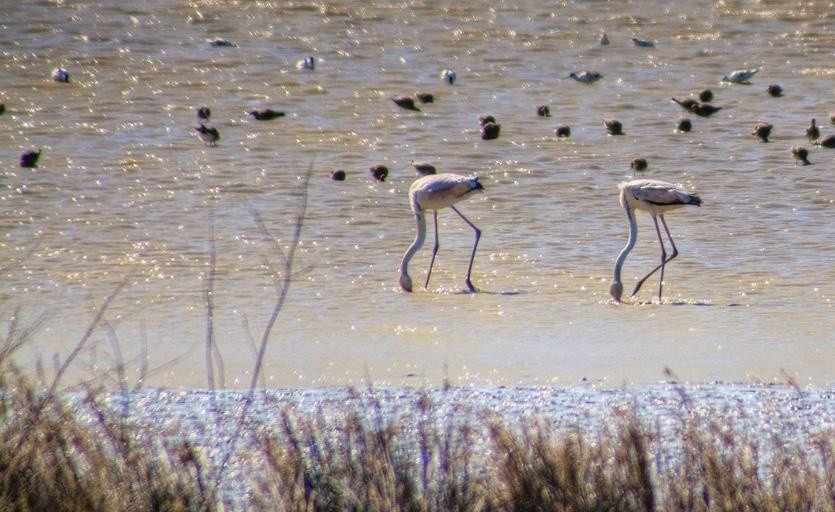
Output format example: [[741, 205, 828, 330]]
[[295, 55, 316, 70], [805, 117, 834, 148], [604, 117, 622, 136], [608, 177, 702, 305], [192, 105, 219, 147], [249, 108, 286, 122], [789, 145, 810, 167], [387, 66, 457, 111], [670, 87, 724, 136], [51, 66, 69, 84], [331, 161, 438, 183], [568, 69, 602, 88], [630, 37, 656, 47], [629, 157, 650, 174], [536, 103, 552, 117], [18, 150, 40, 166], [475, 113, 503, 141], [398, 171, 484, 294], [766, 84, 784, 97], [752, 123, 774, 143], [720, 69, 758, 86], [553, 125, 572, 137]]
